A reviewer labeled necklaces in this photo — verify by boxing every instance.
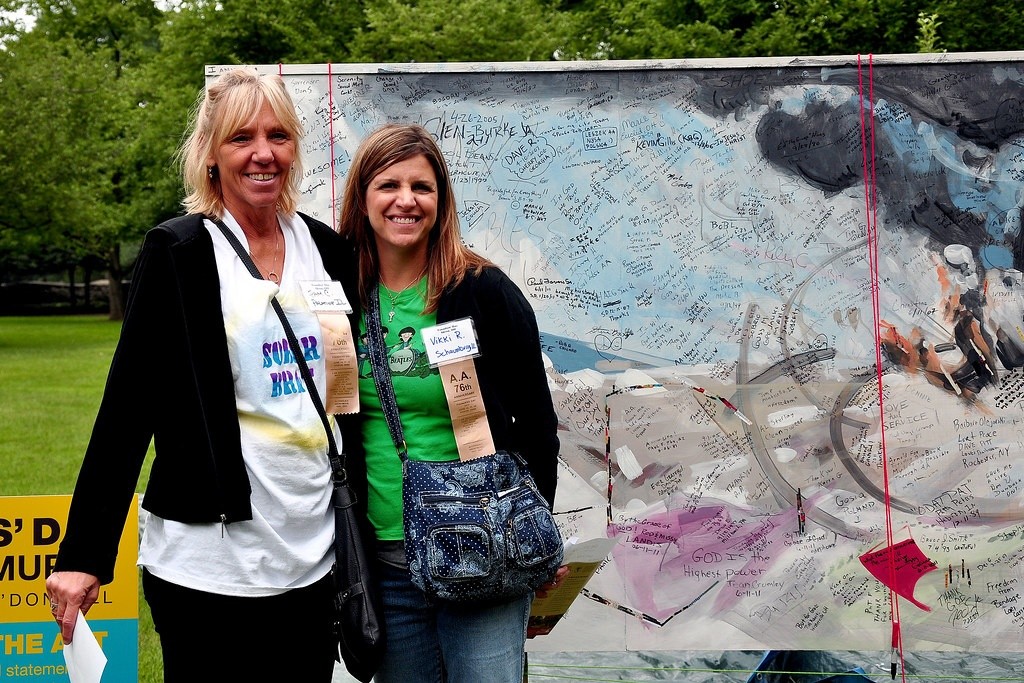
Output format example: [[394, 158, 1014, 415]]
[[249, 222, 279, 284], [379, 264, 428, 322]]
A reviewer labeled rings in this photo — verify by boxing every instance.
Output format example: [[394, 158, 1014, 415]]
[[51, 602, 58, 612]]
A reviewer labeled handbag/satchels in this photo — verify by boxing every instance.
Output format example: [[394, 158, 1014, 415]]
[[401, 451, 567, 613], [330, 453, 386, 683]]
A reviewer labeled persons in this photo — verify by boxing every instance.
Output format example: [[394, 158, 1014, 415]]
[[337, 122, 569, 683], [45, 70, 364, 683]]
